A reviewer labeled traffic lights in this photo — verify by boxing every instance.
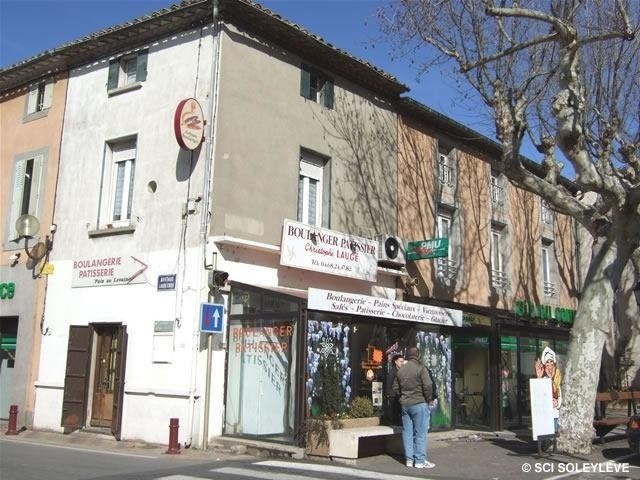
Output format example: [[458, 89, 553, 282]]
[[209, 269, 229, 289]]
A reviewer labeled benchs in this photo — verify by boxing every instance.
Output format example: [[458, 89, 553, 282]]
[[593, 392, 640, 444], [329, 425, 406, 459]]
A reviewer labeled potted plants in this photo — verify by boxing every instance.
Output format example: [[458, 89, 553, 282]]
[[305, 395, 382, 456]]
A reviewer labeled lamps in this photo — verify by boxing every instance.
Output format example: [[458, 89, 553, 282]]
[[15, 215, 57, 262]]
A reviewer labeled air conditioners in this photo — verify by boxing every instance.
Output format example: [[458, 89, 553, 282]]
[[373, 233, 407, 269]]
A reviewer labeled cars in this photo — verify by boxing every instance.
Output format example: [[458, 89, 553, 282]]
[[626, 413, 640, 454]]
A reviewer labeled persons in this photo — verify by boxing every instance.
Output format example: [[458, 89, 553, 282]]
[[384, 355, 404, 424], [392, 346, 437, 468]]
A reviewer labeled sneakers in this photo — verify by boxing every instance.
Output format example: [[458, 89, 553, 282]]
[[406, 458, 414, 467], [414, 459, 436, 469]]
